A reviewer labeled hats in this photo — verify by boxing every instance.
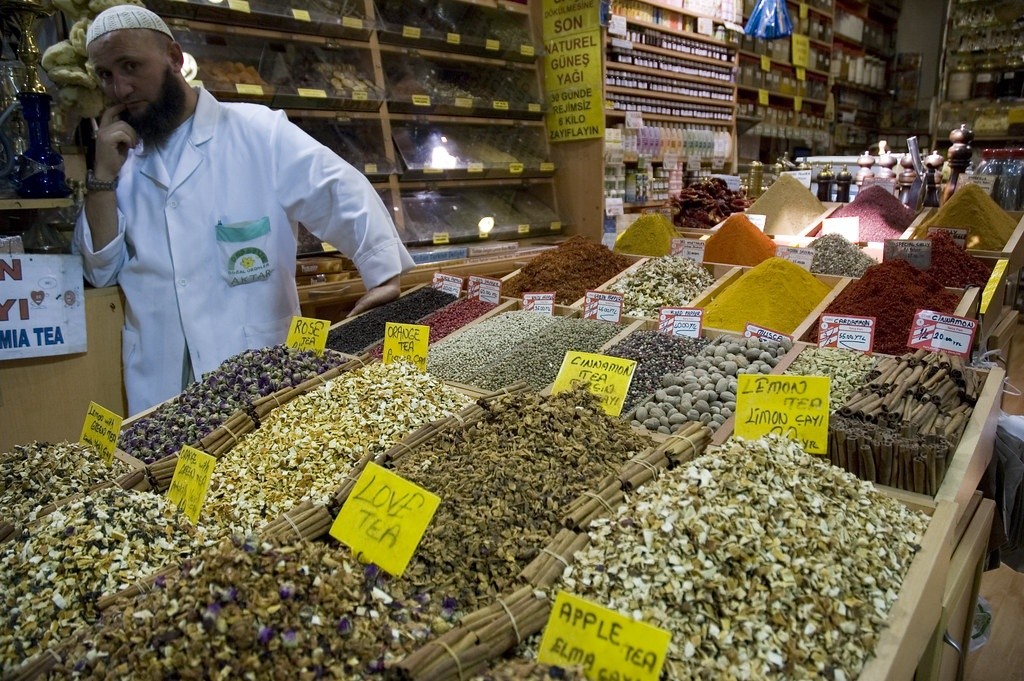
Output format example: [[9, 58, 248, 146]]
[[86, 5, 175, 49]]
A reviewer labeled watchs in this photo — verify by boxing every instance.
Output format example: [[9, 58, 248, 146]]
[[85, 170, 120, 192]]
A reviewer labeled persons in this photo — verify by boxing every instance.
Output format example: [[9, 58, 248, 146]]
[[72, 4, 417, 418]]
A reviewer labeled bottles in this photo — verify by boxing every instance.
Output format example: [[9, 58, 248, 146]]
[[606, 28, 735, 120], [15, 91, 72, 197], [746, 124, 974, 207]]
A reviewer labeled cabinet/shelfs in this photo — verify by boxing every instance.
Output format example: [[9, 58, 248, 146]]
[[1, 1, 1022, 456]]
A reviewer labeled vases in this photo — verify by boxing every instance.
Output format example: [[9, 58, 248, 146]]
[[10, 91, 67, 199]]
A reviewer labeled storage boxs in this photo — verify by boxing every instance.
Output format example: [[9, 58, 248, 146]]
[[113, 199, 1024, 680]]
